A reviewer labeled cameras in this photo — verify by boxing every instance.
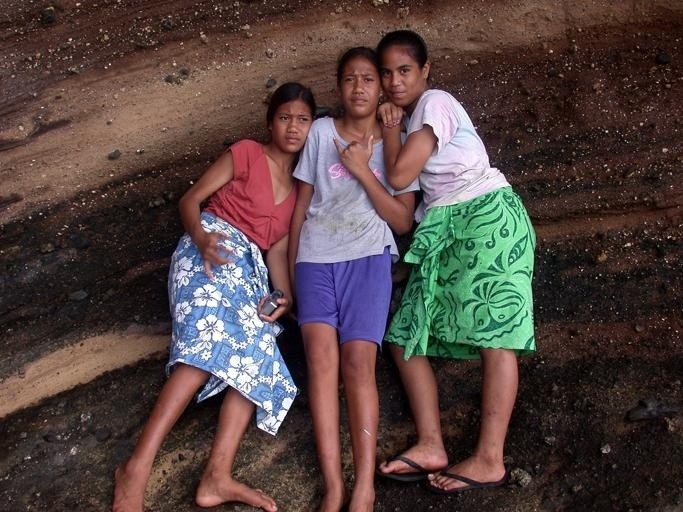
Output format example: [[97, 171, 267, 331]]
[[263, 289, 283, 316]]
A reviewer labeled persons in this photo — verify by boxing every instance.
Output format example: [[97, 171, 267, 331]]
[[374, 28, 536, 494], [108, 80, 319, 512], [286, 45, 421, 510]]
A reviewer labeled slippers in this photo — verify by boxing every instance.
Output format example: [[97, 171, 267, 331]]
[[376, 455, 506, 493]]
